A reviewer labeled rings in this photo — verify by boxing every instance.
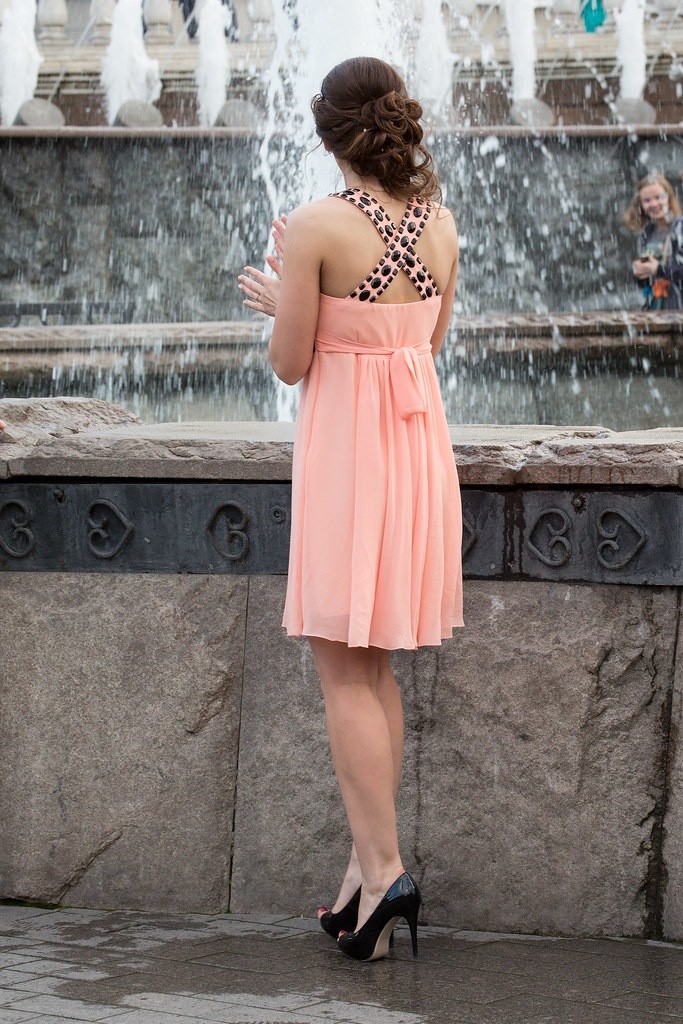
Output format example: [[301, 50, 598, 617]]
[[256, 294, 260, 300]]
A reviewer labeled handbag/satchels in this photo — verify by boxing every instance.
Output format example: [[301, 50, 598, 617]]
[[641, 277, 683, 312]]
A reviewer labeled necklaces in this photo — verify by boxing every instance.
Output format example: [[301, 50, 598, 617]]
[[353, 185, 385, 192]]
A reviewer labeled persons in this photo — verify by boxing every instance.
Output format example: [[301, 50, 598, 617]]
[[624, 174, 683, 311], [180, 0, 239, 43], [238, 56, 466, 962]]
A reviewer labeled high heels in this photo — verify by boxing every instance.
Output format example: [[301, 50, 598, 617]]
[[317, 880, 394, 944], [337, 871, 421, 962]]
